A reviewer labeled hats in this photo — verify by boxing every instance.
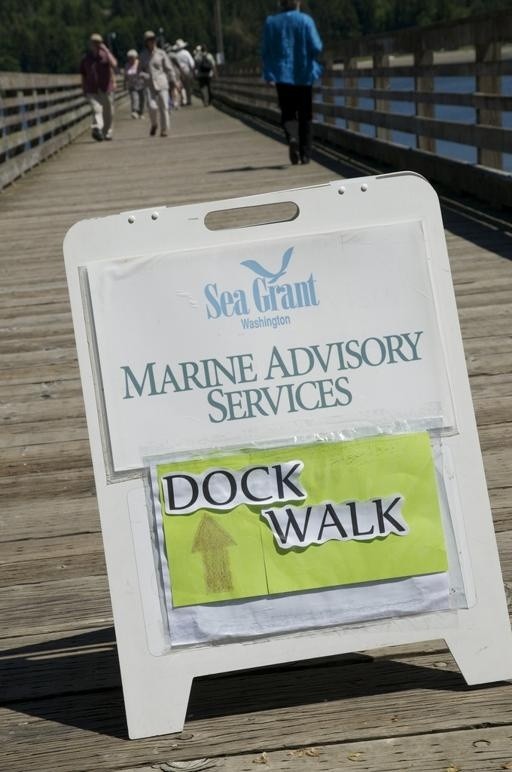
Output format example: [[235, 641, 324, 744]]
[[172, 38, 187, 50], [143, 31, 155, 39], [89, 33, 103, 42]]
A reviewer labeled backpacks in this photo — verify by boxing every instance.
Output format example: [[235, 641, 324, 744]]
[[200, 53, 213, 72]]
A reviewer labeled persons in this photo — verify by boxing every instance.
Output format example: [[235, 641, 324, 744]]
[[259, 0, 327, 168], [136, 30, 180, 138], [123, 38, 217, 120], [78, 32, 120, 141]]
